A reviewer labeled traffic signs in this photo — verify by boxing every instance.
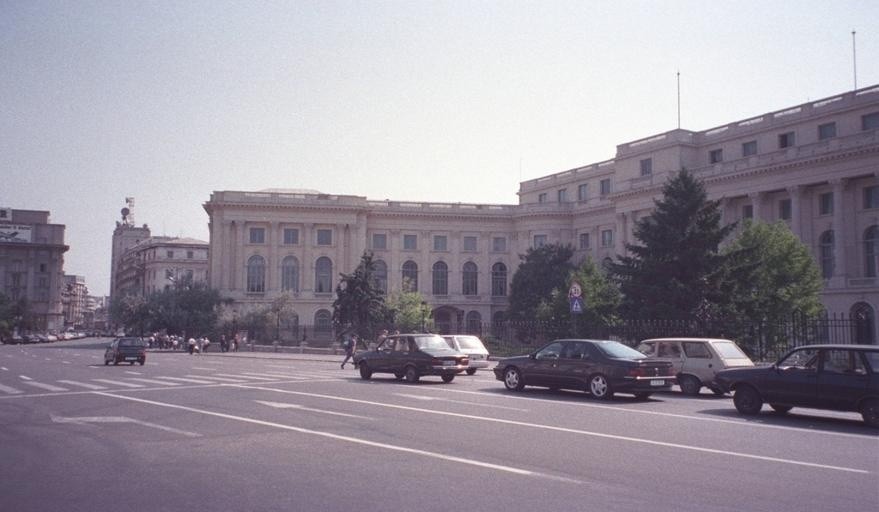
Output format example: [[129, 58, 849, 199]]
[[570, 282, 582, 298]]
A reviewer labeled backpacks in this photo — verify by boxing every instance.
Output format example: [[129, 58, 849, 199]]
[[344, 339, 353, 353]]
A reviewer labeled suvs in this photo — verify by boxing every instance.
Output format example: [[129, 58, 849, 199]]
[[441, 335, 489, 376], [104, 336, 145, 367]]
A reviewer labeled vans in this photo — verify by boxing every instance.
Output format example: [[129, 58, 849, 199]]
[[635, 338, 756, 395]]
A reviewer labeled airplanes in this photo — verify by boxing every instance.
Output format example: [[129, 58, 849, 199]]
[[0, 231, 19, 239]]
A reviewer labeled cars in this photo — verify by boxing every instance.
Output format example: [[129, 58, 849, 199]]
[[493, 339, 676, 401], [353, 333, 469, 385], [712, 344, 879, 431], [15, 328, 90, 343]]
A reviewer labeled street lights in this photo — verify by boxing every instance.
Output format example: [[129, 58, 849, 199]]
[[168, 275, 178, 290], [231, 308, 238, 335], [275, 306, 281, 343]]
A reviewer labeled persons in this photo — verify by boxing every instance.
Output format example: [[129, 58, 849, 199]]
[[147, 329, 244, 355], [392, 329, 401, 351], [376, 330, 389, 351], [340, 334, 361, 370]]
